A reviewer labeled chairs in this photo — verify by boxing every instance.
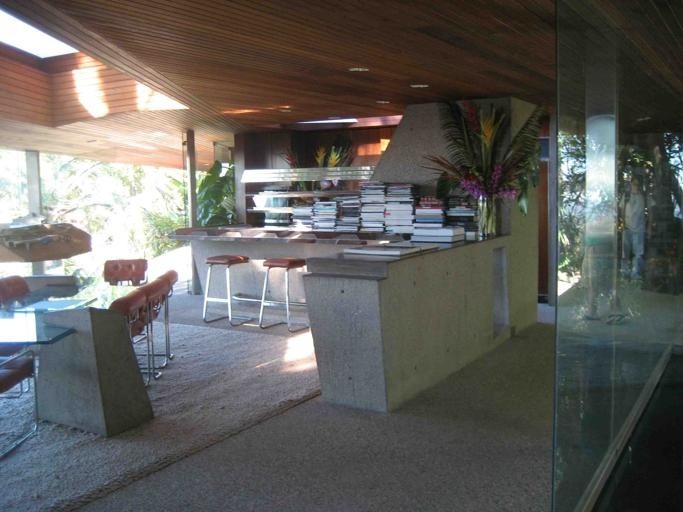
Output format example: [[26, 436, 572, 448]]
[[0, 258, 178, 460]]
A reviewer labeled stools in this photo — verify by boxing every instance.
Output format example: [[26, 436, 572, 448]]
[[202, 254, 307, 332]]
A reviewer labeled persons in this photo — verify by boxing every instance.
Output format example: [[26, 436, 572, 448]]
[[618, 174, 652, 285], [580, 171, 628, 322]]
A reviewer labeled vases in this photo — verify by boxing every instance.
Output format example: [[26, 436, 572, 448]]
[[473, 188, 501, 241]]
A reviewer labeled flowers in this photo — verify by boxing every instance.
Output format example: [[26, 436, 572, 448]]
[[432, 97, 564, 199]]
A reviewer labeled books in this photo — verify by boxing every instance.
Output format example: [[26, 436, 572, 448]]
[[250, 178, 484, 244], [343, 245, 439, 256]]
